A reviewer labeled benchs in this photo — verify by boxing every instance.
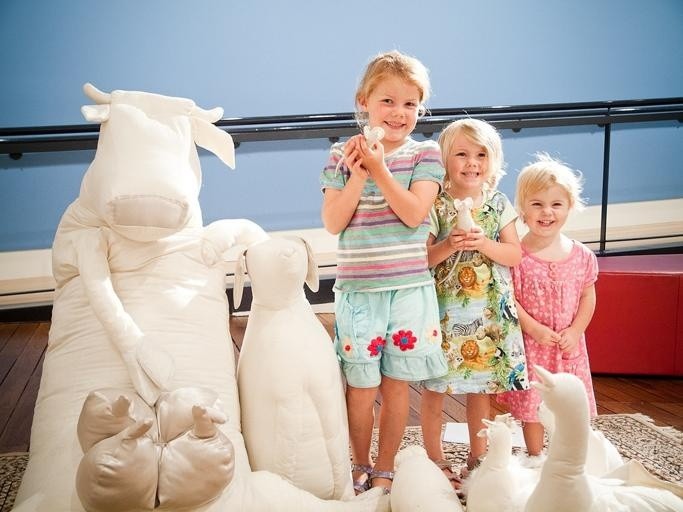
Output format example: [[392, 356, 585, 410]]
[[584, 254, 683, 380]]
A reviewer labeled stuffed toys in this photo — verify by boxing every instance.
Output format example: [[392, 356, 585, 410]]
[[437, 195, 475, 285], [10, 81, 387, 512], [389, 361, 683, 512], [231, 232, 356, 499], [334, 125, 384, 176]]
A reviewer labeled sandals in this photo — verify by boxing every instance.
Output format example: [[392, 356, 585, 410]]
[[463, 452, 484, 480], [350, 464, 374, 496], [370, 469, 394, 495], [434, 458, 468, 506]]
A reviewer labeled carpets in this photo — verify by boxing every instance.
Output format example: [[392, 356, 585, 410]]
[[0, 413, 683, 512]]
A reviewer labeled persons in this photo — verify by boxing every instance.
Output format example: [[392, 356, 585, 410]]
[[495, 151, 599, 455], [419, 118, 531, 492], [321, 49, 448, 496]]
